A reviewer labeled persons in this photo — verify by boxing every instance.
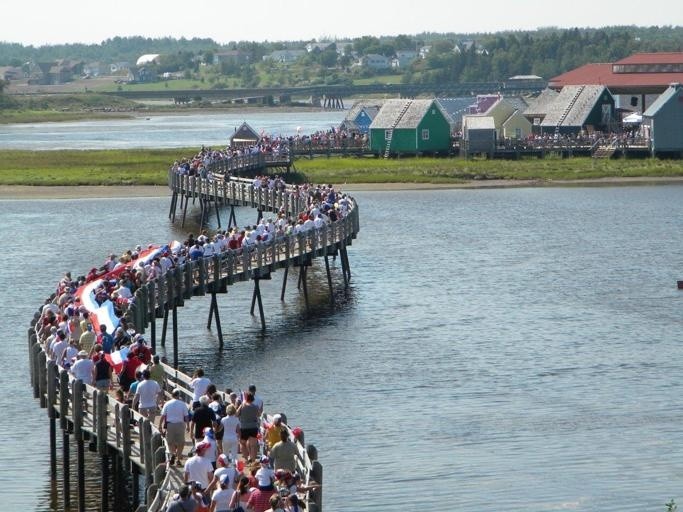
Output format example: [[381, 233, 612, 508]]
[[37, 175, 348, 511], [224, 170, 231, 183], [170, 129, 369, 180], [527, 129, 643, 149], [451, 128, 463, 147]]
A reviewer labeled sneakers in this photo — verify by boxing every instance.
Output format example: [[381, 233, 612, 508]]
[[177, 460, 181, 467], [169, 452, 176, 468]]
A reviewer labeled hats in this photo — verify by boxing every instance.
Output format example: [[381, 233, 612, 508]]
[[216, 453, 229, 466], [257, 470, 270, 487], [196, 441, 211, 450], [219, 474, 228, 485], [185, 479, 201, 488]]
